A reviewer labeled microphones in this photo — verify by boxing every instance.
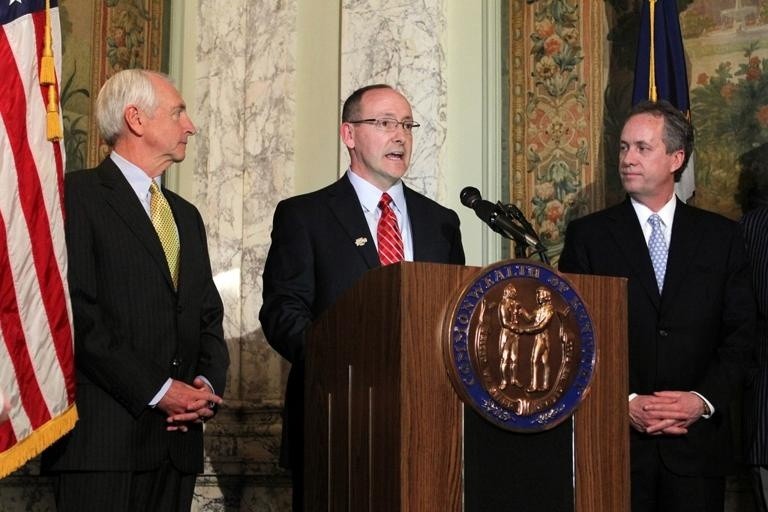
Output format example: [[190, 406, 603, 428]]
[[459, 185, 544, 250]]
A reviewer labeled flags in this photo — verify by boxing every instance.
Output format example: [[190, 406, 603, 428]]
[[631, 0, 696, 209], [0, 0, 83, 480]]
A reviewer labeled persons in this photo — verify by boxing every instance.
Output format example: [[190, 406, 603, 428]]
[[59, 70, 230, 512], [557, 101, 755, 511], [259, 84, 467, 512], [498, 283, 556, 393]]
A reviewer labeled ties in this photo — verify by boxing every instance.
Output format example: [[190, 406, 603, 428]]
[[147, 177, 182, 292], [645, 213, 670, 295], [374, 192, 406, 268]]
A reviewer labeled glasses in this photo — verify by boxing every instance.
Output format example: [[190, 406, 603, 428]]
[[346, 117, 422, 134]]
